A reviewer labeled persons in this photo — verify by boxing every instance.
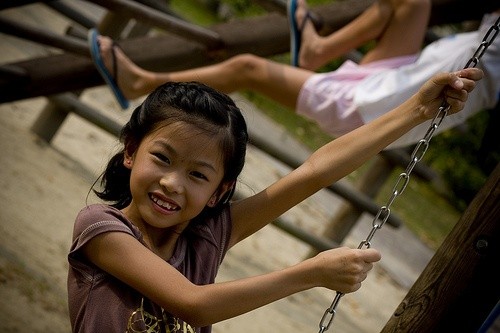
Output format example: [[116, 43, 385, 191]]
[[86, 0, 500, 152], [65, 67, 486, 333]]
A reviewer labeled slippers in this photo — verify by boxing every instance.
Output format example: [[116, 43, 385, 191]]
[[87, 28, 131, 112], [286, 0, 317, 66]]
